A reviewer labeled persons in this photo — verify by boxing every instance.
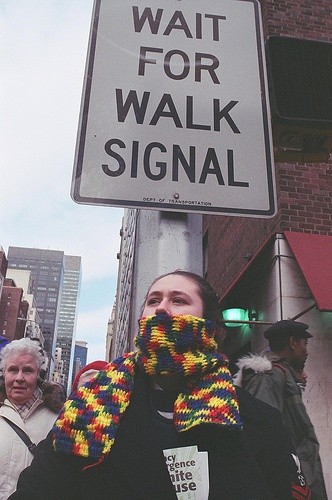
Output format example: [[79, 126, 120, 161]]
[[232, 320, 328, 500], [7, 269, 314, 500], [0, 338, 66, 500]]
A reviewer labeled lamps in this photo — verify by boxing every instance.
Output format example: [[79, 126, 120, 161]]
[[222, 304, 246, 327]]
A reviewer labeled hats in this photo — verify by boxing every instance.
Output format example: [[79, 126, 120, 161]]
[[263, 320, 314, 341]]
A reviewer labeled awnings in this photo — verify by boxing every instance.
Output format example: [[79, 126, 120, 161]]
[[219, 234, 275, 309], [284, 231, 332, 311]]
